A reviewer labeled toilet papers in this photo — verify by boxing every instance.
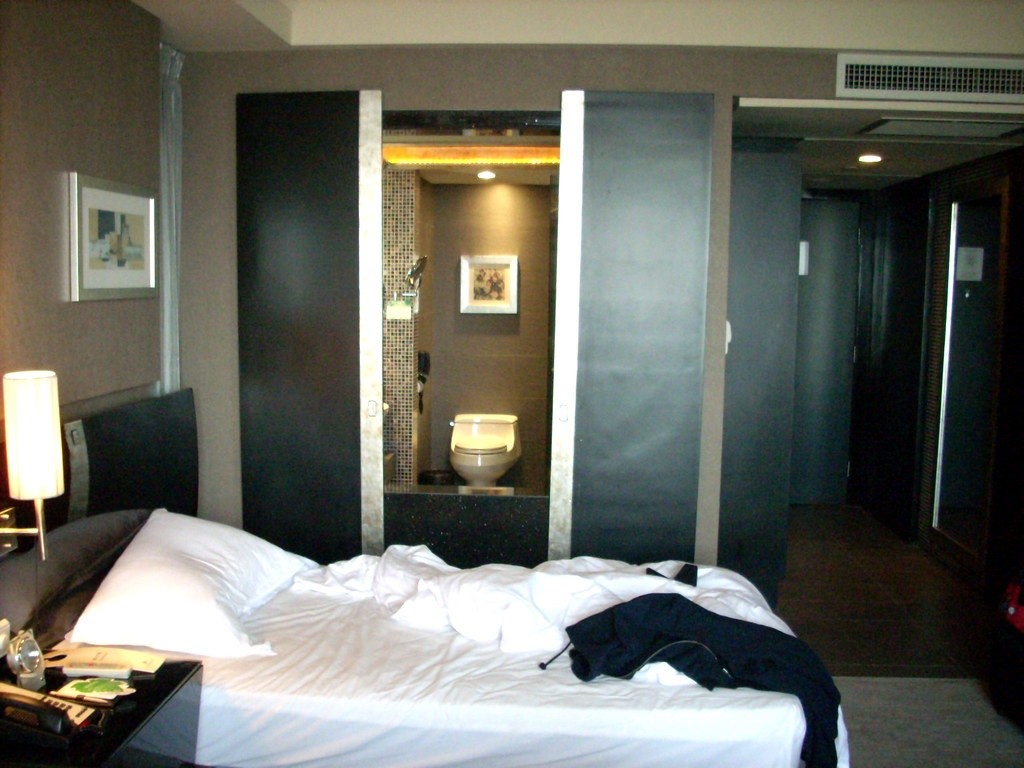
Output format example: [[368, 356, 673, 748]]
[[417, 380, 424, 393]]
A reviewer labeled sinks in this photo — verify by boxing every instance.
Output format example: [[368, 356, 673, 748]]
[[383, 402, 389, 417]]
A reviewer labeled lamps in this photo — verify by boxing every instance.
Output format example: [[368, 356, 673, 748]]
[[0, 370, 65, 560]]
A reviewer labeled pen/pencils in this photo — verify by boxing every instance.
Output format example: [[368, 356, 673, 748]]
[[50, 690, 108, 704]]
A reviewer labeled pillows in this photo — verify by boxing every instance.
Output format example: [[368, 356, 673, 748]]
[[65, 508, 322, 656]]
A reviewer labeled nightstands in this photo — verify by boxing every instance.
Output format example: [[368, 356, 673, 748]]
[[0, 650, 204, 768]]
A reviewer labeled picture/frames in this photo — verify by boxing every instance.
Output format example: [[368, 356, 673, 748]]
[[69, 173, 159, 302], [460, 255, 518, 314]]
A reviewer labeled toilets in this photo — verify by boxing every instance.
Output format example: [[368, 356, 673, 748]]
[[448, 413, 522, 488]]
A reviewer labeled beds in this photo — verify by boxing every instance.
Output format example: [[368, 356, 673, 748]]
[[0, 508, 850, 768]]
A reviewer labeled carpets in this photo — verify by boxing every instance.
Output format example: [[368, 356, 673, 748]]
[[831, 677, 1024, 767]]
